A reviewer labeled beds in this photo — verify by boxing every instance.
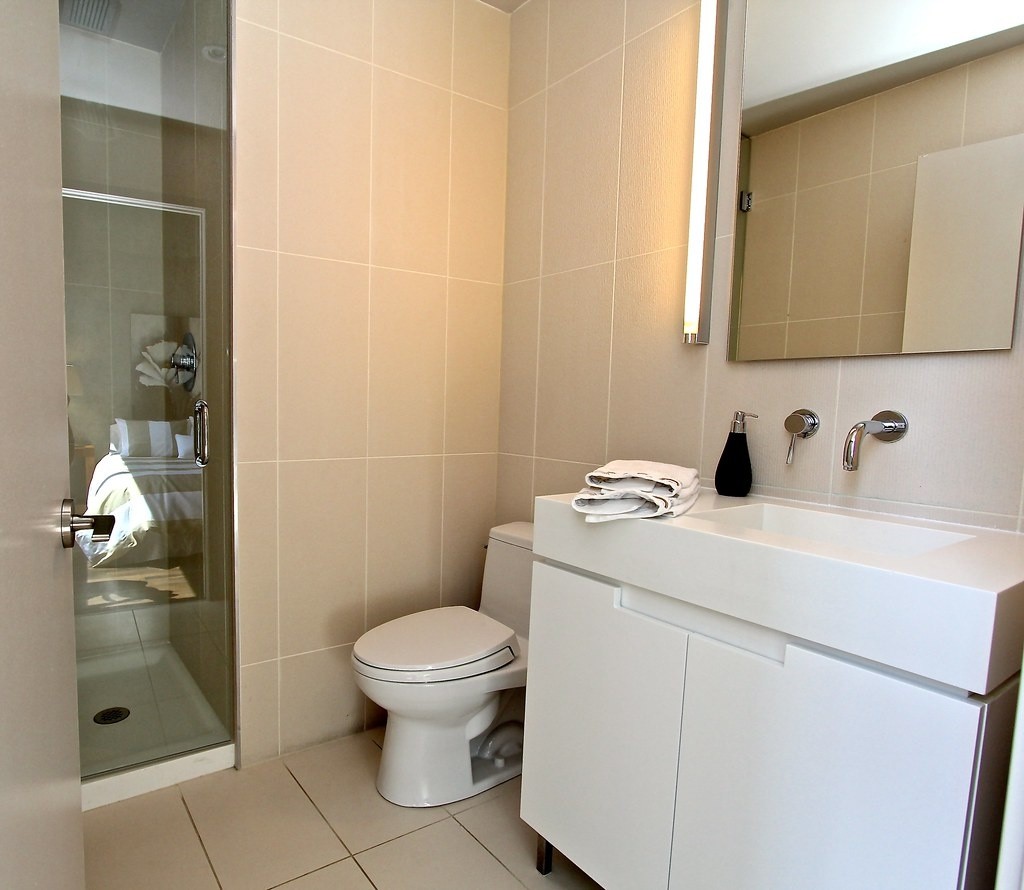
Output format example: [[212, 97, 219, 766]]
[[74, 421, 205, 567]]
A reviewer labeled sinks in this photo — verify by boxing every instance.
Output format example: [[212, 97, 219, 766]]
[[679, 502, 978, 563]]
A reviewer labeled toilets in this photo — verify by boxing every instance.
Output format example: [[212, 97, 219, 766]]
[[349, 520, 535, 808]]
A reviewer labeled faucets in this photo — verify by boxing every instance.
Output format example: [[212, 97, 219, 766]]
[[842, 408, 909, 472]]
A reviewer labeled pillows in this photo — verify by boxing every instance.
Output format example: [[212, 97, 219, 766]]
[[115, 418, 188, 458], [174, 433, 196, 461]]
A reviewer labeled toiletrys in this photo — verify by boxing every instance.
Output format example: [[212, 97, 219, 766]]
[[715, 410, 759, 497]]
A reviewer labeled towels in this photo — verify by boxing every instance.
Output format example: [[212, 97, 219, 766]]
[[571, 458, 702, 525]]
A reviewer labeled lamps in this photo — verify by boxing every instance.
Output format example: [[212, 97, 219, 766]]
[[679, 26, 726, 344]]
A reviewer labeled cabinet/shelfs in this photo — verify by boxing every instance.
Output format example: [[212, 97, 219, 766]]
[[520, 564, 1022, 889]]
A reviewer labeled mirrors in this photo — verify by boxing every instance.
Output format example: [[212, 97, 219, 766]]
[[723, 39, 1023, 363]]
